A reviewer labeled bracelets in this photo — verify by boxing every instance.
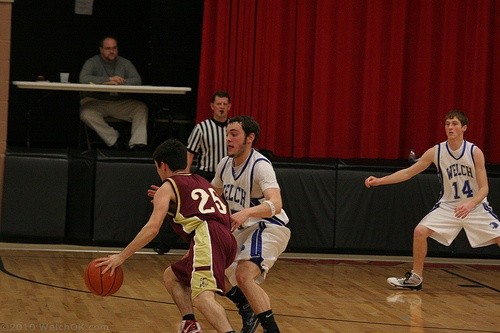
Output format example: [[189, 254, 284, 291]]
[[262, 201, 275, 218]]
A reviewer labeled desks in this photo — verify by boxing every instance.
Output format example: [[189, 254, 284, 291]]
[[11, 80, 191, 152]]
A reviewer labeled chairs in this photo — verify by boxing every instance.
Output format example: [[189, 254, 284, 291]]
[[24, 73, 194, 155]]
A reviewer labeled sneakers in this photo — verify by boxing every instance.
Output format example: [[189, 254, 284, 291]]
[[387, 293, 422, 302], [241, 312, 260, 333], [387, 270, 422, 291], [179, 320, 201, 333]]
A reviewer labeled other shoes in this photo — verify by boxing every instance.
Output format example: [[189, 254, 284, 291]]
[[111, 137, 122, 156]]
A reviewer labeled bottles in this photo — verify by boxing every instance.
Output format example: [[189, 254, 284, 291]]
[[409, 148, 415, 162]]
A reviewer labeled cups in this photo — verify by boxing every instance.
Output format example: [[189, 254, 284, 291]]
[[60, 73, 69, 83]]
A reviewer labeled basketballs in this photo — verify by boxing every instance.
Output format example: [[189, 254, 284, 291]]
[[84, 259, 123, 296]]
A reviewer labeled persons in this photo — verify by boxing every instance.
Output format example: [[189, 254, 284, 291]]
[[365, 109, 500, 290], [186, 91, 231, 182], [94, 139, 237, 333], [79, 36, 148, 156], [209, 115, 291, 333]]
